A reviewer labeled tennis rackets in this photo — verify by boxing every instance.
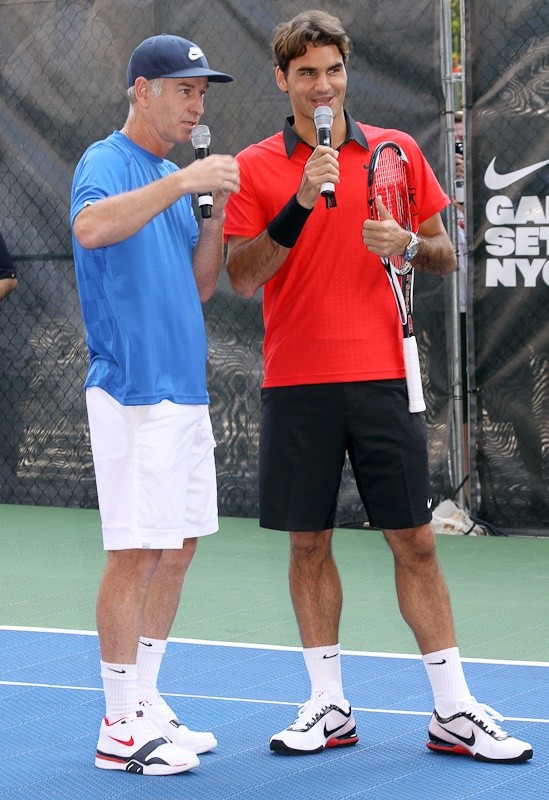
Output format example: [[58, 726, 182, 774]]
[[366, 139, 429, 415]]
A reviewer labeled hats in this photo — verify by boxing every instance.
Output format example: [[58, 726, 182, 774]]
[[126, 31, 234, 88]]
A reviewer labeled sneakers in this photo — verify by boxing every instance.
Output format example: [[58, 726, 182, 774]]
[[268, 687, 359, 754], [424, 697, 533, 763], [140, 698, 218, 754], [95, 715, 198, 775]]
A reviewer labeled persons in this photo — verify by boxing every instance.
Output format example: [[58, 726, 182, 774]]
[[70, 34, 241, 775], [223, 10, 532, 762]]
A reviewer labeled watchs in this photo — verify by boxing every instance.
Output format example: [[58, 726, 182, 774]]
[[404, 230, 420, 261]]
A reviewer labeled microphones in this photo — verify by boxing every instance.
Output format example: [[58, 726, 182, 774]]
[[314, 106, 337, 209], [191, 125, 212, 219]]
